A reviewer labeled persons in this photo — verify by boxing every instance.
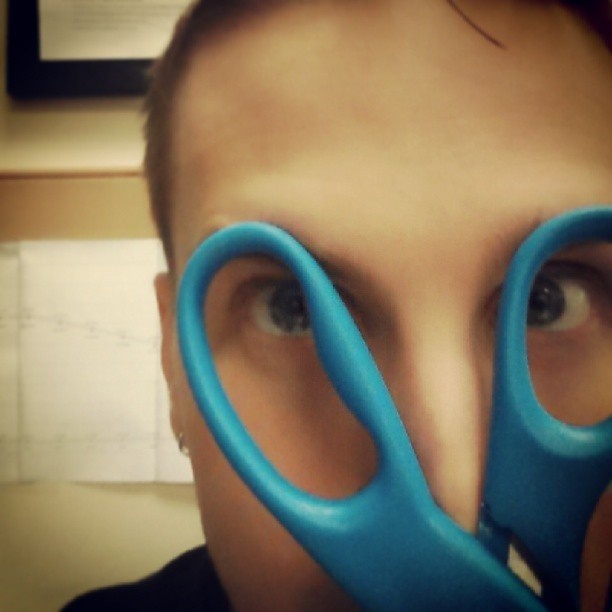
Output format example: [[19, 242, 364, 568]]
[[53, 0, 611, 612]]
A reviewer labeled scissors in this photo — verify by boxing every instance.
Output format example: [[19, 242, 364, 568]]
[[175, 206, 610, 612]]
